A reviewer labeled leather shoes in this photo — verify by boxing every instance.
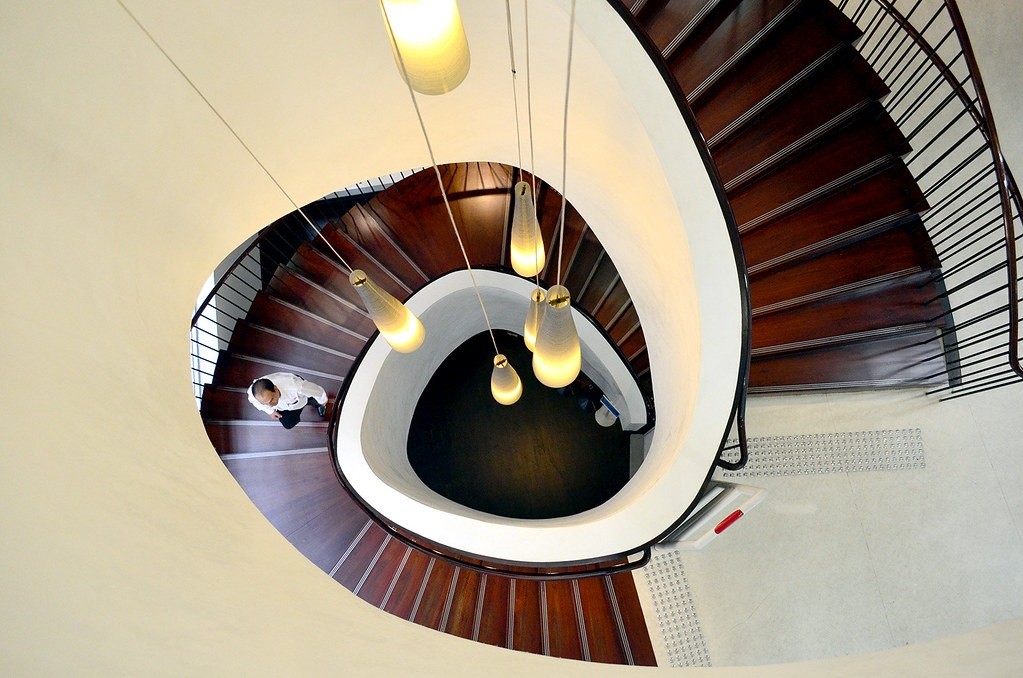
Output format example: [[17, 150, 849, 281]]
[[318, 404, 327, 417]]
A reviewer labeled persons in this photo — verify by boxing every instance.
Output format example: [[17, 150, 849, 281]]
[[247, 372, 328, 430]]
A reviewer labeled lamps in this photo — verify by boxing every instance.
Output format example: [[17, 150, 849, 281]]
[[110, 0, 582, 405]]
